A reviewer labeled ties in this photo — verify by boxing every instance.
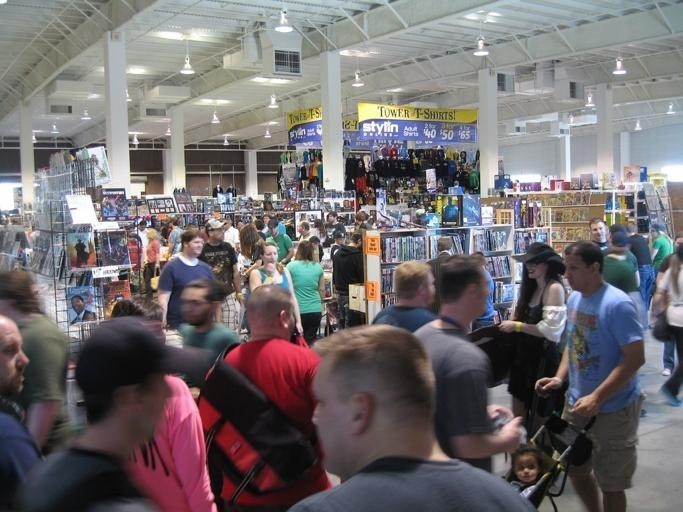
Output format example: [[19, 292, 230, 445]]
[[76, 317, 81, 321]]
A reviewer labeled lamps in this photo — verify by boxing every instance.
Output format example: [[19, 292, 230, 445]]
[[585, 94, 595, 107], [667, 104, 676, 114], [274, 11, 292, 32], [473, 22, 488, 56], [29, 89, 277, 146], [634, 120, 642, 131], [180, 40, 195, 75], [612, 48, 627, 75], [351, 70, 365, 87]]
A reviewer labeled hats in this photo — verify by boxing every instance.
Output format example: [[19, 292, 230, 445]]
[[511, 242, 566, 274], [333, 230, 345, 238], [205, 220, 227, 231], [649, 220, 666, 230], [77, 316, 211, 386]]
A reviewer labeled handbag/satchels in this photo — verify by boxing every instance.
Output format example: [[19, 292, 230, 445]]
[[150, 276, 160, 290], [653, 312, 675, 341]]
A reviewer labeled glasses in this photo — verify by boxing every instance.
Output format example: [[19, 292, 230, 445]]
[[523, 260, 546, 266]]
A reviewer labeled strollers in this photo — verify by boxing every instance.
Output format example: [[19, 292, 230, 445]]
[[501, 383, 597, 510]]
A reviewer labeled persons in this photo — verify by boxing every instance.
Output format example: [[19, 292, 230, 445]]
[[352, 160, 369, 195], [2, 213, 647, 512], [454, 180, 464, 194], [588, 214, 682, 407], [212, 185, 223, 198], [226, 184, 237, 197]]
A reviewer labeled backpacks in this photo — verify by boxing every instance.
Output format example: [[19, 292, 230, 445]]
[[197, 343, 320, 495]]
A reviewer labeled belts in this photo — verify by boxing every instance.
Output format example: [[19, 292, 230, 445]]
[[638, 264, 652, 268]]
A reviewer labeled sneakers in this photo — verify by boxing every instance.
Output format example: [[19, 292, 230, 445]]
[[661, 369, 671, 375], [660, 385, 681, 406]]
